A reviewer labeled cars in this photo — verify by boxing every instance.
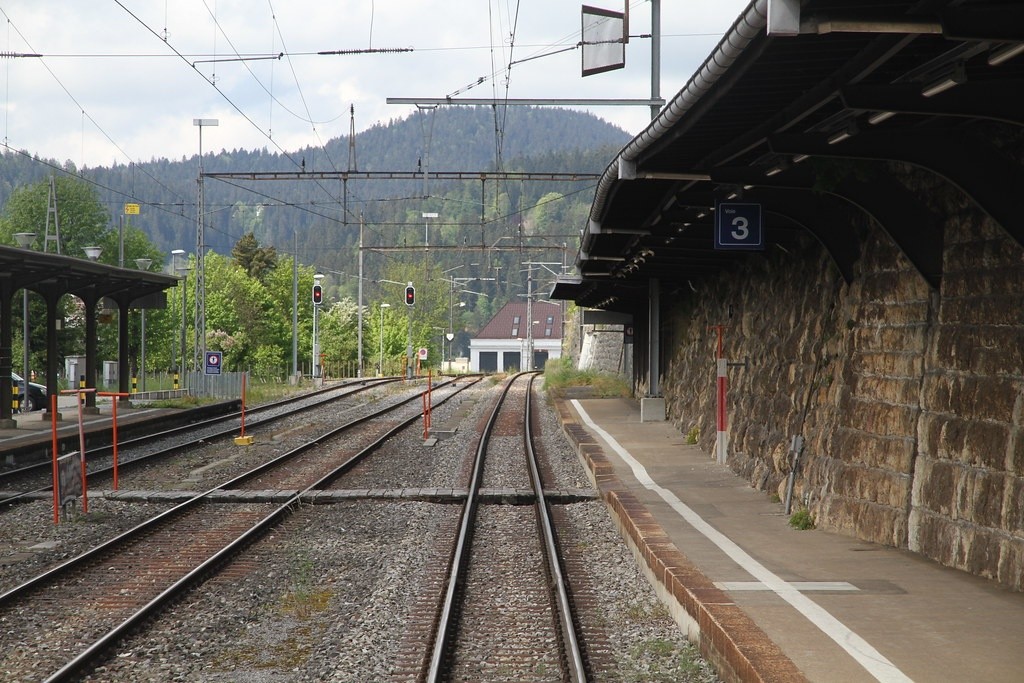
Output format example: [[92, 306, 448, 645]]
[[11, 371, 48, 415]]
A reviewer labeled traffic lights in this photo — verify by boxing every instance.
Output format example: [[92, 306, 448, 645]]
[[405, 286, 416, 305], [312, 285, 322, 305]]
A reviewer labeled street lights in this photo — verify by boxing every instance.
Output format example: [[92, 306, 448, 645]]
[[133, 257, 153, 398], [12, 231, 37, 415], [312, 273, 325, 378], [378, 303, 391, 376], [169, 249, 187, 399]]
